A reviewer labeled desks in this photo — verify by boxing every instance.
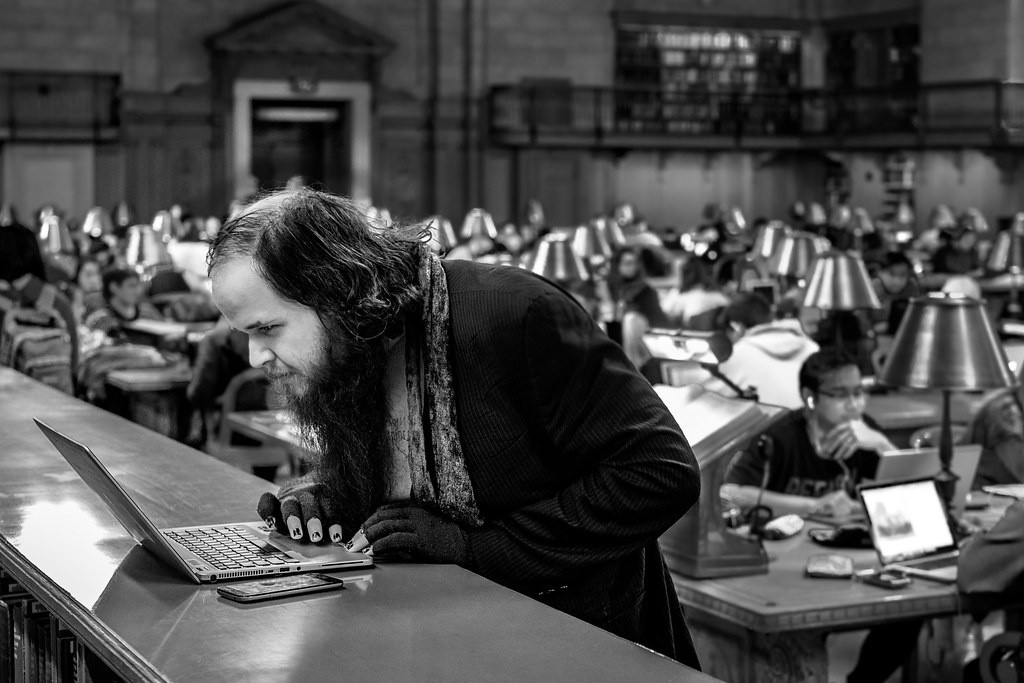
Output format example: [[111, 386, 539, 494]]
[[0, 364, 724, 683], [670, 497, 1024, 683], [868, 392, 982, 449], [106, 366, 194, 443], [225, 410, 322, 464]]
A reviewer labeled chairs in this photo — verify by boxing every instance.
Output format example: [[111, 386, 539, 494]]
[[910, 424, 972, 449], [201, 370, 286, 482]]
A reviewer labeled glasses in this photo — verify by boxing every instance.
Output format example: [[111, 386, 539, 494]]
[[816, 384, 866, 398]]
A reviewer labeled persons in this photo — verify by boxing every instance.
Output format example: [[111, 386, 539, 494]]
[[958, 358, 1024, 489], [870, 254, 921, 316], [184, 317, 268, 446], [0, 203, 48, 282], [608, 247, 667, 327], [701, 292, 822, 410], [956, 496, 1024, 683], [937, 230, 980, 275], [720, 350, 925, 683], [208, 188, 701, 674], [86, 270, 163, 331]]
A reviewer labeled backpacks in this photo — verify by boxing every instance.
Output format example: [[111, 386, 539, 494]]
[[0, 285, 74, 402]]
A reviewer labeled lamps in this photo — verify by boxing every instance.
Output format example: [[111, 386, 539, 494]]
[[875, 292, 1016, 540], [760, 220, 792, 258], [988, 233, 1024, 274], [590, 215, 627, 250], [766, 237, 816, 288], [532, 234, 589, 291], [639, 328, 746, 400], [423, 217, 457, 254], [801, 252, 882, 316], [573, 223, 611, 266], [460, 209, 497, 240]]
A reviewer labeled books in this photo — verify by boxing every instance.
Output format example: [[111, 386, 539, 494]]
[[651, 384, 768, 461]]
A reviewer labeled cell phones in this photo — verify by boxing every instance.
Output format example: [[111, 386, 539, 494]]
[[216, 573, 344, 602]]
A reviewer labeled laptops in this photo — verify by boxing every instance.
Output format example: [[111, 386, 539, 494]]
[[32, 417, 373, 583], [855, 476, 963, 584]]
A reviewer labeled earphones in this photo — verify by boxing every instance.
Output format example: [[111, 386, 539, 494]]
[[807, 396, 816, 410]]
